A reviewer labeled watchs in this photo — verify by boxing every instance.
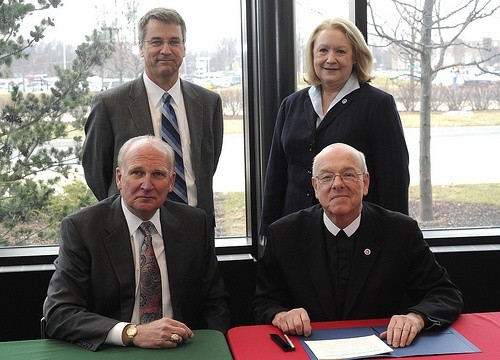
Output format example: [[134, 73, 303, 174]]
[[125, 323, 139, 347]]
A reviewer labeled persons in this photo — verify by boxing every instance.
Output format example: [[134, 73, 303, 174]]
[[450, 67, 469, 85], [252, 143, 463, 348], [82, 8, 223, 242], [256, 17, 410, 249], [43, 135, 231, 352]]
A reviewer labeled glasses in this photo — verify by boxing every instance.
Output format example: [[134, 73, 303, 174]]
[[316, 171, 364, 185], [143, 38, 183, 47]]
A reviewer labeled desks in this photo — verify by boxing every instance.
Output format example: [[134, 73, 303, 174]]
[[0, 329, 232, 360], [226, 312, 500, 360]]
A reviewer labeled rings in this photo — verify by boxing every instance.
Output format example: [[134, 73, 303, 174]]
[[169, 333, 180, 342]]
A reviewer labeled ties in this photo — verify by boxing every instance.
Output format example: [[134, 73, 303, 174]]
[[137, 221, 163, 325], [161, 92, 188, 205]]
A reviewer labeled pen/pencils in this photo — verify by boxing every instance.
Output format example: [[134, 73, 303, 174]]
[[284, 333, 295, 349]]
